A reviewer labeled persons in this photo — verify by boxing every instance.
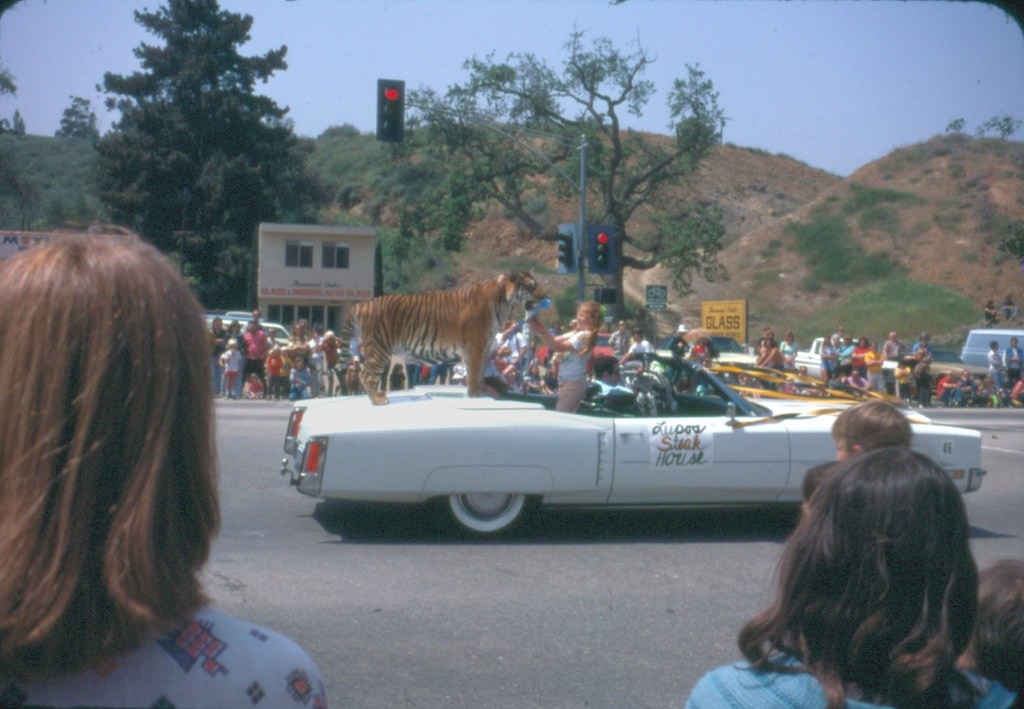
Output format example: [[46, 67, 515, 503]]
[[1001, 296, 1018, 321], [983, 300, 1000, 326], [204, 310, 467, 402], [682, 399, 1024, 709], [0, 224, 328, 709], [820, 327, 1024, 409], [483, 302, 820, 414]]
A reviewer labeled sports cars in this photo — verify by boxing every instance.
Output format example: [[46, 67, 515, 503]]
[[277, 347, 988, 543]]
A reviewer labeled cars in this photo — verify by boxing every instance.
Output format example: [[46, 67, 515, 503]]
[[209, 310, 291, 347], [903, 347, 989, 388], [795, 338, 901, 385]]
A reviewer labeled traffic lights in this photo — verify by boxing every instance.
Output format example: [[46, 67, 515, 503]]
[[588, 224, 619, 274], [376, 76, 404, 142], [594, 286, 618, 306], [555, 223, 585, 276]]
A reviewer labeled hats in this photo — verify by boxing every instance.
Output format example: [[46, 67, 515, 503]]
[[228, 319, 242, 327], [677, 324, 688, 332], [920, 333, 929, 341]]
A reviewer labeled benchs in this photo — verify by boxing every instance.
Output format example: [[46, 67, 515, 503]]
[[574, 381, 643, 418]]
